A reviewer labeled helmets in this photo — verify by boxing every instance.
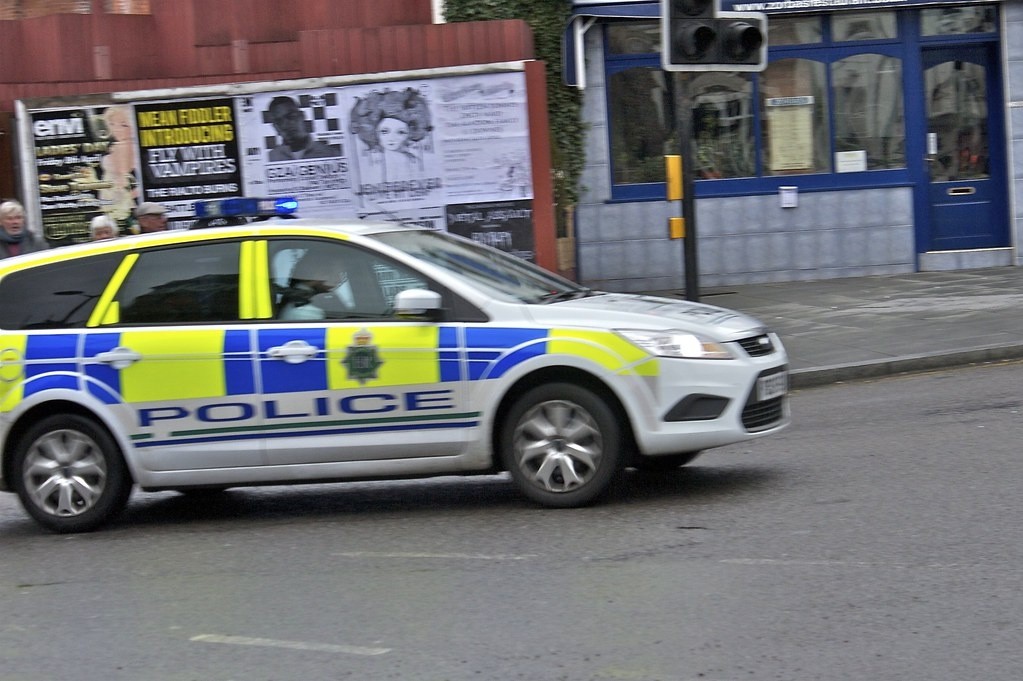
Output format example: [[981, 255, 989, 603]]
[[271, 247, 356, 316]]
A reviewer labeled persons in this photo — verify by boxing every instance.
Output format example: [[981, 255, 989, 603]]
[[0, 200, 51, 260], [134, 202, 168, 234], [89, 215, 120, 241], [279, 246, 350, 320], [266, 96, 343, 162]]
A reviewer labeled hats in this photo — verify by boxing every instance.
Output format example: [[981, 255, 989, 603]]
[[135, 202, 172, 217]]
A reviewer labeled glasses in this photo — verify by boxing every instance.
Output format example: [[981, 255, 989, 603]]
[[142, 215, 166, 221]]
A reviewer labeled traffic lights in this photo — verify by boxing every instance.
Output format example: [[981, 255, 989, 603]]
[[663, 3, 772, 72]]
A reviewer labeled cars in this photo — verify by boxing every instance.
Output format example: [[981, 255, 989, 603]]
[[0, 196, 793, 535]]
